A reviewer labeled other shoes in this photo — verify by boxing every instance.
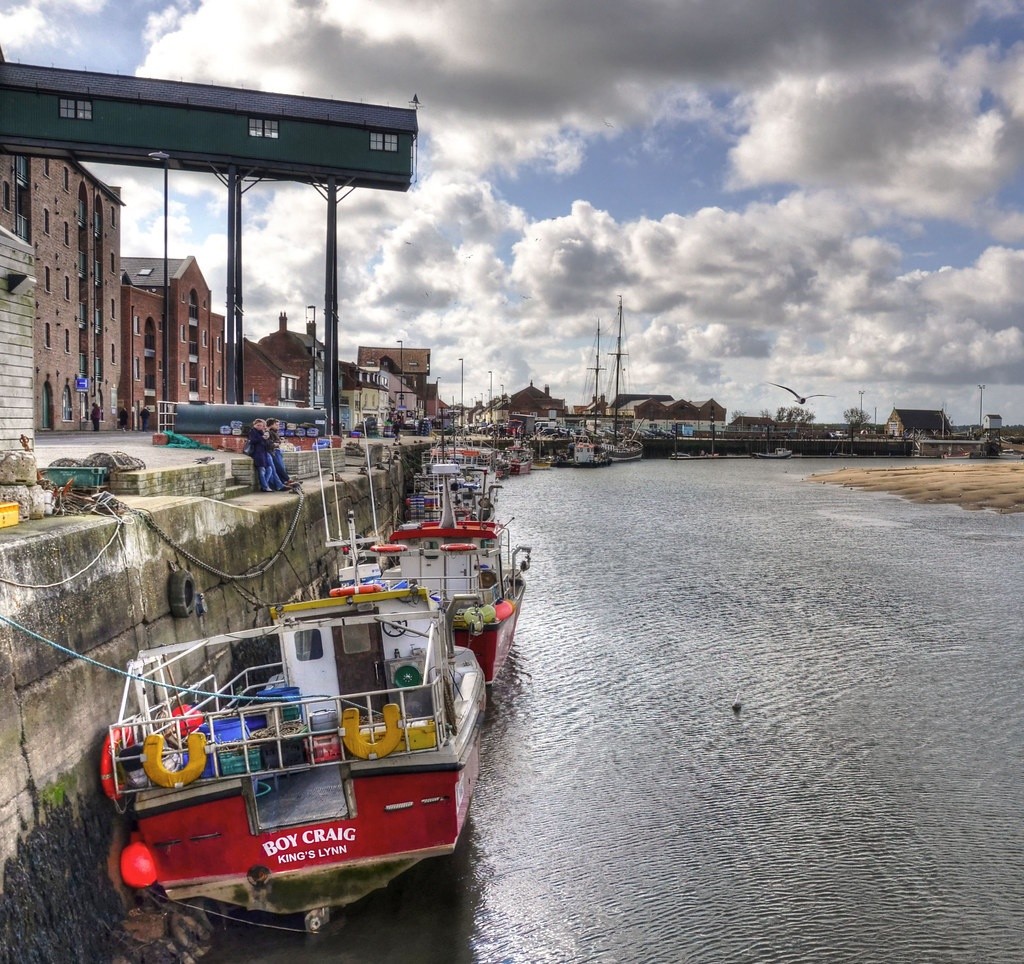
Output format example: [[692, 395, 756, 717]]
[[261, 486, 272, 491], [277, 485, 291, 491]]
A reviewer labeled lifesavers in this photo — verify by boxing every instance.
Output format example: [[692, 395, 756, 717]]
[[399, 524, 422, 530], [341, 702, 402, 761], [607, 459, 612, 466], [100, 727, 138, 801], [440, 542, 478, 552], [370, 544, 408, 553], [166, 568, 197, 618], [141, 732, 208, 789], [328, 583, 383, 598]]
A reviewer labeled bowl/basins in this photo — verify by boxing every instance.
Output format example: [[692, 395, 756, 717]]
[[255, 781, 272, 804]]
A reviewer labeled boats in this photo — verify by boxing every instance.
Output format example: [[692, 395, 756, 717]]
[[261, 296, 643, 688], [944, 451, 971, 459], [999, 448, 1024, 459], [103, 408, 487, 936]]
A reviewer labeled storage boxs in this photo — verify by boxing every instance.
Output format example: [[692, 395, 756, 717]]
[[359, 719, 437, 754], [304, 732, 350, 764], [219, 748, 262, 776], [181, 751, 221, 779], [37, 467, 108, 487], [0, 501, 19, 528], [261, 737, 307, 770]]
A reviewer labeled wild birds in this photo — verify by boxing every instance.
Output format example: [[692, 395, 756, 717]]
[[765, 381, 836, 404], [732, 693, 742, 710]]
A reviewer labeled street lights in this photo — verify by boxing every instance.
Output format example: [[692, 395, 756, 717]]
[[308, 304, 316, 406], [500, 384, 505, 417], [146, 149, 171, 416], [459, 357, 465, 428], [751, 448, 793, 458], [397, 340, 404, 421], [978, 384, 986, 432], [858, 391, 866, 427], [488, 371, 493, 421]]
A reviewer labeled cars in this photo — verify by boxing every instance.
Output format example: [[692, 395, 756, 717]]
[[619, 427, 645, 439], [647, 428, 674, 439], [829, 430, 849, 439], [641, 429, 655, 439]]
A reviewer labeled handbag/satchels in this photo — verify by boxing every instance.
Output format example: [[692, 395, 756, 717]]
[[244, 429, 254, 455]]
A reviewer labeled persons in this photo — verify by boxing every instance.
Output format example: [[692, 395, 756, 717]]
[[538, 426, 543, 432], [558, 429, 563, 438], [120, 408, 128, 432], [90, 403, 100, 431], [517, 422, 526, 440], [393, 421, 402, 446], [140, 407, 150, 432], [785, 431, 791, 439], [248, 418, 303, 492], [802, 429, 809, 439], [491, 424, 504, 439], [904, 429, 910, 438]]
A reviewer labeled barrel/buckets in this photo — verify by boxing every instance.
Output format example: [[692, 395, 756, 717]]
[[256, 687, 302, 724], [464, 598, 515, 625], [198, 718, 258, 779]]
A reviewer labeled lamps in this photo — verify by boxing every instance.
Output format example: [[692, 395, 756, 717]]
[[8, 273, 37, 295]]
